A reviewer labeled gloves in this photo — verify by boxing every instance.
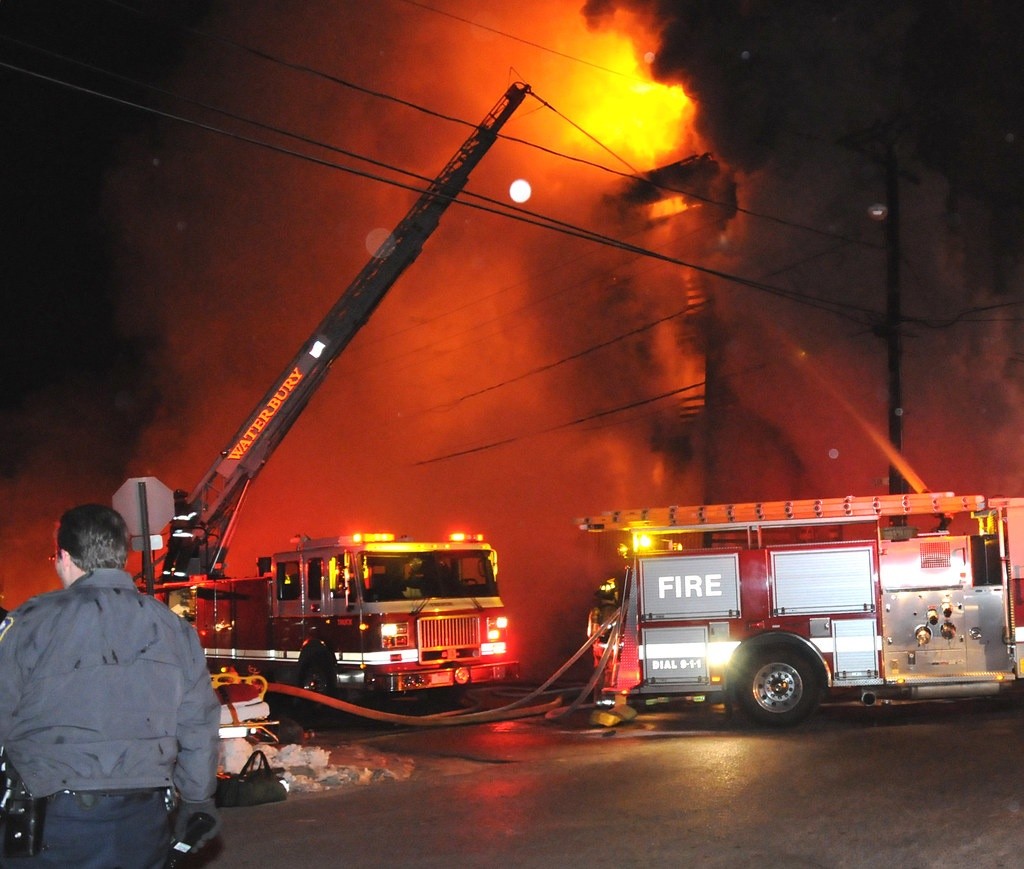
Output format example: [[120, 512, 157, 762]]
[[175, 798, 223, 854]]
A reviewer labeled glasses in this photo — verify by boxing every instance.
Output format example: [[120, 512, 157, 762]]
[[49, 552, 57, 561]]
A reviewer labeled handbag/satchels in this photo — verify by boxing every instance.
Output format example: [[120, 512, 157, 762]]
[[215, 750, 289, 807], [3, 795, 45, 858]]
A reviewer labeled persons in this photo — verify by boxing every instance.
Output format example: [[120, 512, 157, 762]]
[[585, 589, 621, 704], [0, 501, 224, 869]]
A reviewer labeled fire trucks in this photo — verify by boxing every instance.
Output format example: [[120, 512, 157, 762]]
[[574, 489, 1024, 726], [155, 532, 524, 724]]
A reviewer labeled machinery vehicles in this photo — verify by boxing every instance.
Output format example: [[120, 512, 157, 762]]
[[131, 74, 542, 744]]
